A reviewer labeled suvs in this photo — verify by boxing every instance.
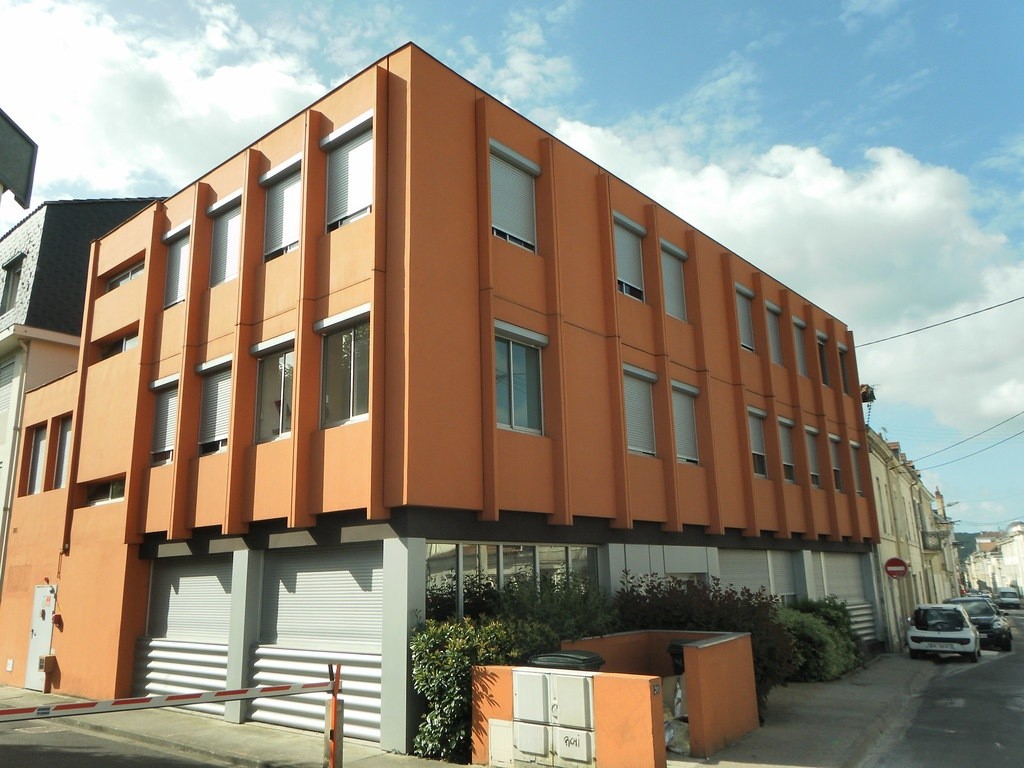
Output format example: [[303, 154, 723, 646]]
[[907, 605, 982, 663]]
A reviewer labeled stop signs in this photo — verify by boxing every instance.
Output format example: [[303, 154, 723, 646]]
[[885, 558, 907, 580]]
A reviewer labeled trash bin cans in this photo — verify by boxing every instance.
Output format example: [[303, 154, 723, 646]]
[[666, 639, 694, 675], [526, 651, 606, 672]]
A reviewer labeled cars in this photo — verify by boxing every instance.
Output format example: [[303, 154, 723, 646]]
[[943, 593, 1013, 654], [996, 588, 1021, 610]]
